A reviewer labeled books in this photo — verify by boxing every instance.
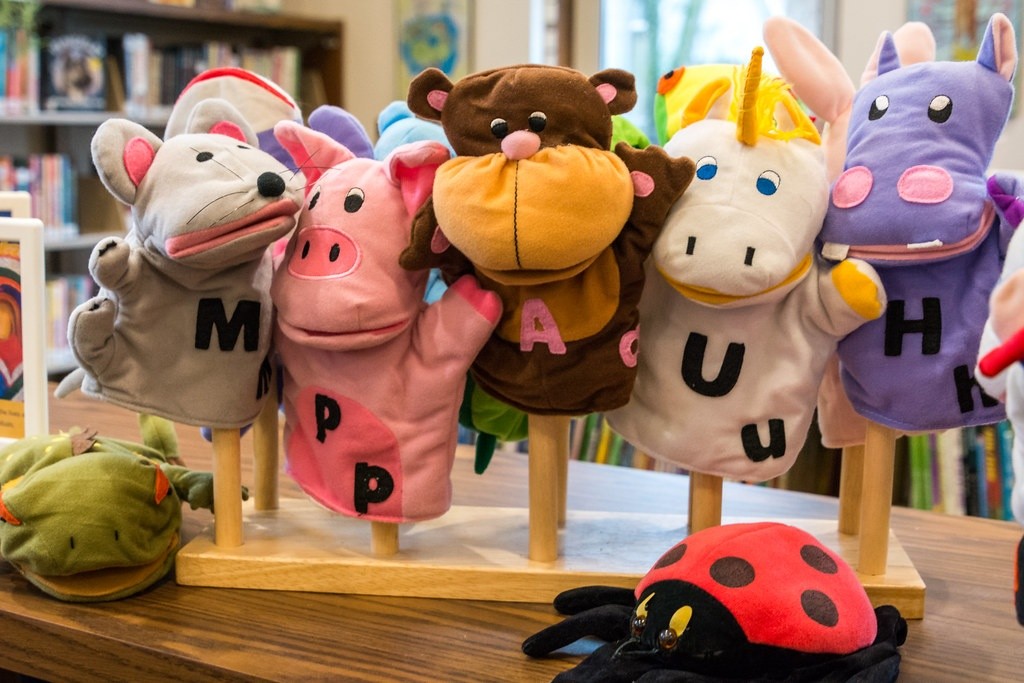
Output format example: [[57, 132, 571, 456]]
[[459, 408, 680, 486], [0, 22, 315, 364], [891, 418, 1017, 523]]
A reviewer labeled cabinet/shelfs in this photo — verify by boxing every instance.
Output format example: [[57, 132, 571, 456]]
[[0, 0, 350, 383]]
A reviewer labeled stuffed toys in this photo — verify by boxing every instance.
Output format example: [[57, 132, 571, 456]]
[[396, 64, 702, 420], [453, 105, 656, 476], [595, 43, 888, 487], [518, 518, 908, 683], [974, 219, 1022, 541], [649, 57, 795, 151], [0, 409, 251, 611], [369, 98, 462, 309], [811, 11, 1022, 435], [152, 65, 375, 449], [266, 116, 500, 527], [56, 99, 291, 429], [759, 14, 944, 452]]
